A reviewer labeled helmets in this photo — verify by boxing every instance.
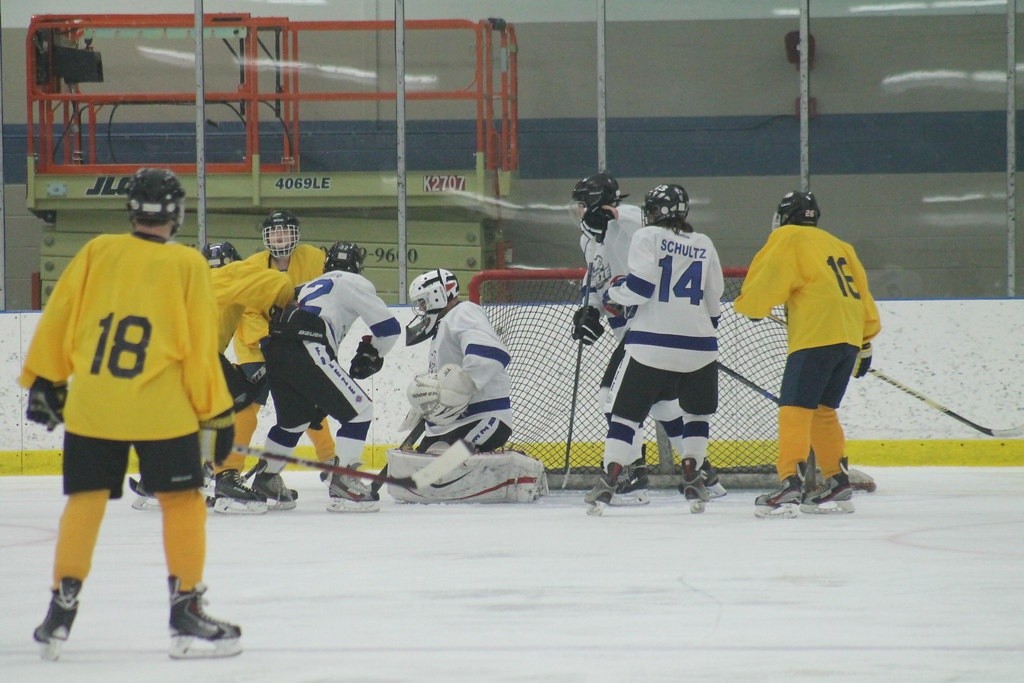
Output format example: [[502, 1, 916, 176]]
[[640, 183, 691, 227], [261, 209, 300, 258], [322, 240, 366, 274], [771, 191, 820, 231], [569, 170, 622, 228], [409, 267, 460, 335], [201, 241, 242, 267], [125, 167, 186, 238]]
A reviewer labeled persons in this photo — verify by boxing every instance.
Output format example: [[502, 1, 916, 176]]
[[733, 190, 882, 518], [124, 210, 549, 514], [17, 168, 247, 661], [571, 174, 727, 517]]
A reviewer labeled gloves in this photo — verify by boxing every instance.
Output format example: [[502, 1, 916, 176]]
[[348, 334, 383, 380], [579, 205, 615, 244], [26, 376, 67, 431], [852, 341, 872, 379], [571, 306, 604, 346]]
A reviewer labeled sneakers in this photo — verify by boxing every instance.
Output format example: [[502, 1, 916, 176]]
[[583, 460, 622, 516], [798, 456, 856, 514], [199, 461, 268, 514], [611, 443, 653, 505], [169, 575, 244, 658], [126, 475, 216, 513], [680, 456, 711, 514], [32, 576, 83, 662], [320, 455, 390, 513], [753, 472, 803, 519], [250, 458, 299, 510], [676, 458, 728, 499]]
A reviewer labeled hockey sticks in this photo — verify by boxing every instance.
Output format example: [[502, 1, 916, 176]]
[[718, 362, 780, 404], [561, 234, 597, 490], [197, 374, 356, 498], [128, 363, 266, 496], [768, 314, 1024, 439], [339, 419, 431, 495], [231, 436, 476, 492]]
